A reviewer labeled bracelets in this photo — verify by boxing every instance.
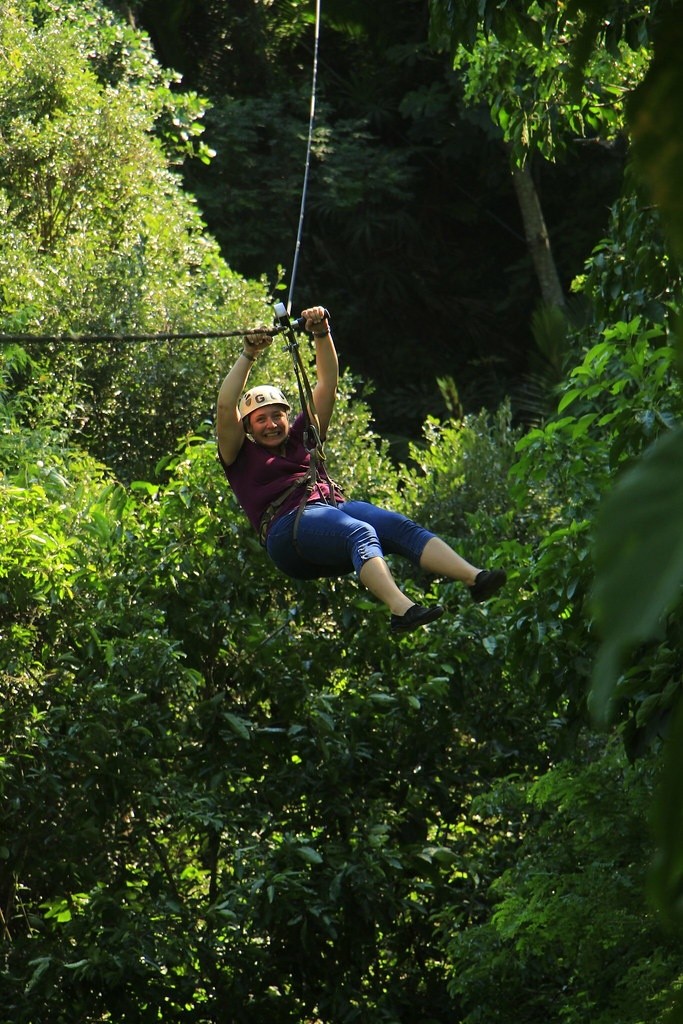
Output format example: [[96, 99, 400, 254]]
[[243, 352, 256, 361], [312, 325, 330, 338]]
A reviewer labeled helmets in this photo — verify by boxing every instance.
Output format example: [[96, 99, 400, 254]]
[[239, 386, 290, 419]]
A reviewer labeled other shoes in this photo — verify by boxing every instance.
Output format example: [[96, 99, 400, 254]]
[[470, 569, 507, 603], [391, 603, 444, 634]]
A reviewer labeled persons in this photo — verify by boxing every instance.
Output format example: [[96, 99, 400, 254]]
[[215, 307, 507, 633]]
